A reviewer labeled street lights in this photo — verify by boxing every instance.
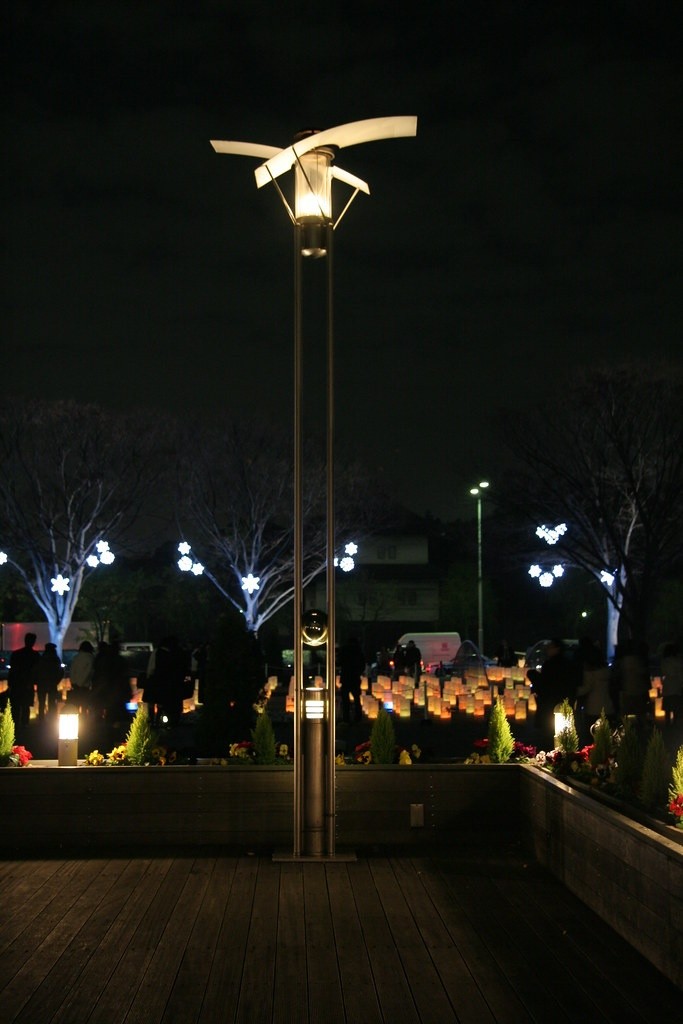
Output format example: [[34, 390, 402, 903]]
[[209, 113, 419, 863], [470, 481, 490, 655]]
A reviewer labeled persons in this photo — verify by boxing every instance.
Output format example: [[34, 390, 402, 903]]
[[0, 632, 683, 749]]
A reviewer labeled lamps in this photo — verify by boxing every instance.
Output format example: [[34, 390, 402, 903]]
[[211, 115, 418, 258], [58, 713, 80, 766]]
